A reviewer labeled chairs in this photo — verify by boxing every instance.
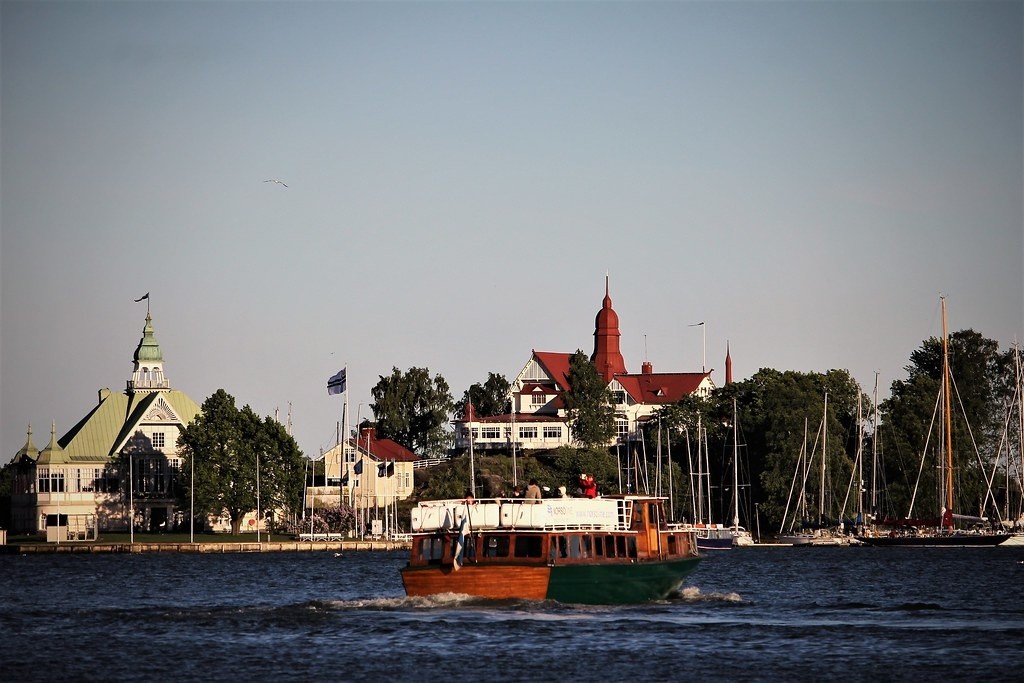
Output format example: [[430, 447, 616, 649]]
[[299, 533, 344, 542]]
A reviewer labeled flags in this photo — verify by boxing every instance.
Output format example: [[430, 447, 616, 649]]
[[327, 368, 347, 395], [134, 293, 148, 302], [453, 506, 470, 571]]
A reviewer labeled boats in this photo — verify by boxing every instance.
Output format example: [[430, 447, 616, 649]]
[[401, 387, 709, 607]]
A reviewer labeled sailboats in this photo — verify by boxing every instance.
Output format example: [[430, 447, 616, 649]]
[[665, 289, 1024, 549]]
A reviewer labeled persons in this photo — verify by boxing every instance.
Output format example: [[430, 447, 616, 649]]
[[558, 486, 568, 498], [511, 486, 525, 504], [858, 524, 1008, 539], [464, 492, 474, 504], [524, 479, 542, 504], [579, 473, 597, 498]]
